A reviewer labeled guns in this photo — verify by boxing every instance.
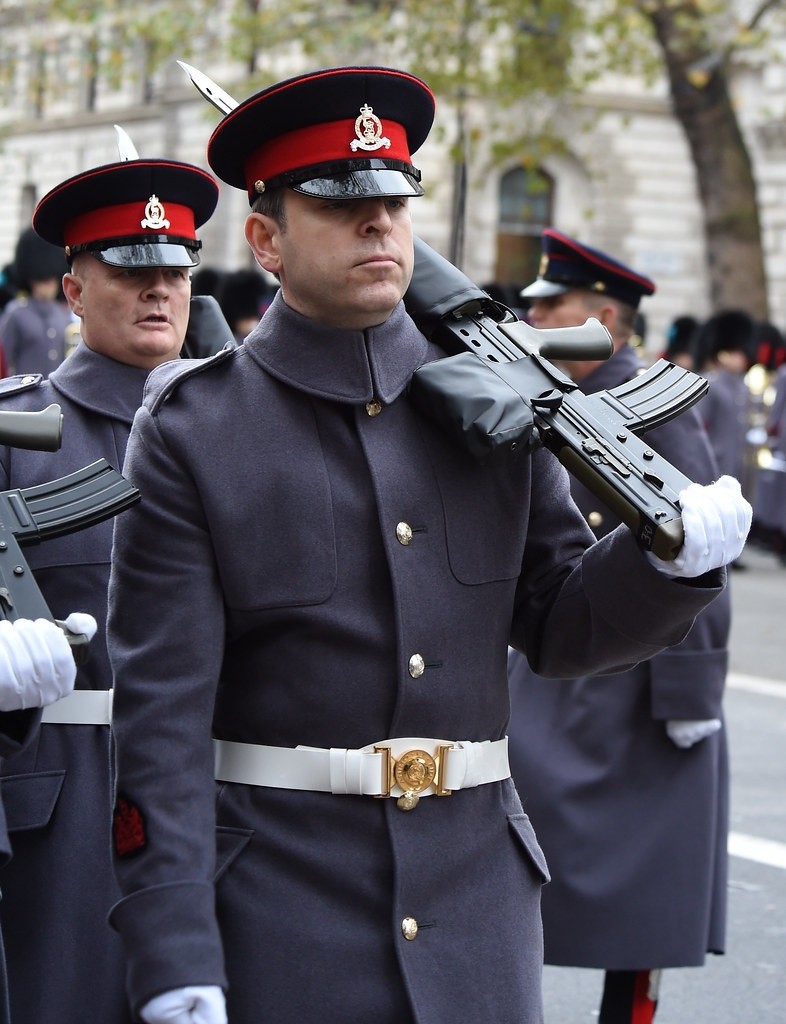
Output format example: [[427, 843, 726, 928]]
[[173, 53, 713, 564], [112, 124, 241, 358], [0, 401, 143, 622]]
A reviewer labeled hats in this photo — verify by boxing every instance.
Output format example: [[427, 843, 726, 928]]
[[671, 311, 785, 373], [194, 272, 271, 331], [206, 64, 439, 201], [0, 229, 70, 317], [518, 228, 658, 309], [33, 158, 218, 270]]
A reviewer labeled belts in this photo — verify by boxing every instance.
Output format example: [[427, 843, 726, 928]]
[[211, 735, 513, 811], [39, 689, 111, 726]]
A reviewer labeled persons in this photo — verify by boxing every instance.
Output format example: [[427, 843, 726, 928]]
[[506, 227, 726, 1024], [103, 65, 753, 1023], [478, 279, 785, 571], [1, 160, 277, 1024]]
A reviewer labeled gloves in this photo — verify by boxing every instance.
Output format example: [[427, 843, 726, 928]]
[[139, 982, 228, 1024], [646, 475, 752, 578], [0, 611, 96, 709], [664, 717, 721, 750]]
